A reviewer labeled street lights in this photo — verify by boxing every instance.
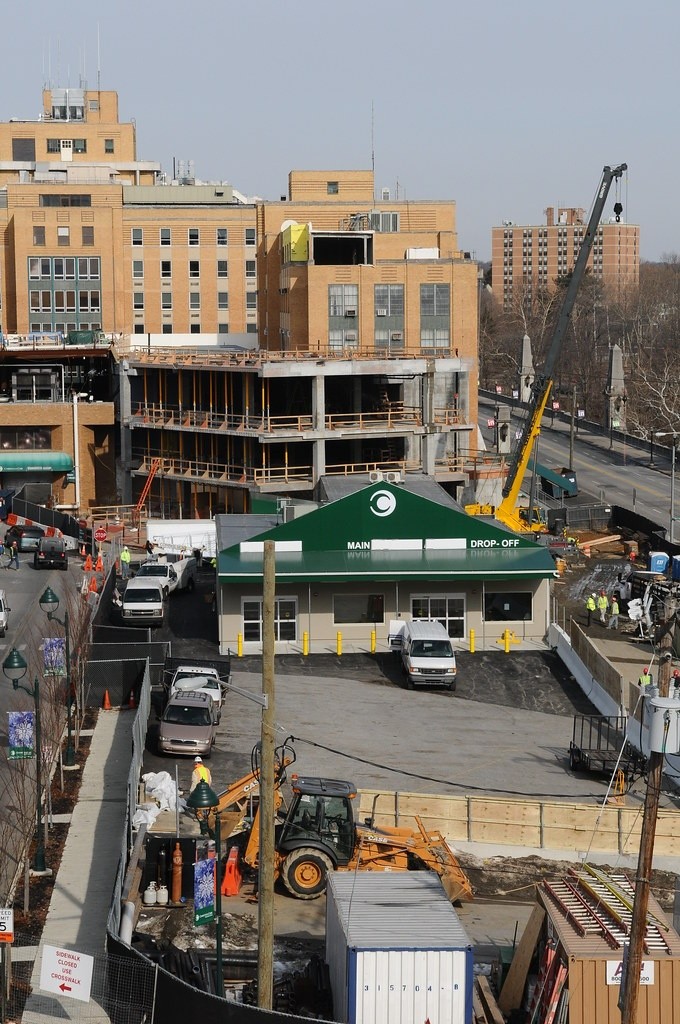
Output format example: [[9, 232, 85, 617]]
[[493, 411, 498, 449], [609, 414, 615, 451], [649, 426, 656, 467], [2, 647, 48, 873], [494, 380, 498, 406], [576, 401, 579, 434], [672, 432, 677, 471], [38, 586, 81, 779], [550, 395, 555, 426], [509, 384, 516, 412], [189, 778, 226, 996]]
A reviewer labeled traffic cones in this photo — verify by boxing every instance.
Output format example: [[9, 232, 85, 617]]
[[81, 543, 87, 556], [104, 689, 113, 711], [127, 689, 138, 710]]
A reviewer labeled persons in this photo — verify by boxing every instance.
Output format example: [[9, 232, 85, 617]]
[[671, 670, 680, 687], [0, 541, 6, 556], [605, 597, 619, 629], [120, 546, 130, 580], [190, 756, 211, 794], [586, 593, 596, 628], [5, 541, 20, 572], [598, 591, 609, 623], [638, 668, 653, 686]]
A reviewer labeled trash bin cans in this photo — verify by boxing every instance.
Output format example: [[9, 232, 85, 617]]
[[526, 937, 567, 1024], [555, 518, 563, 536]]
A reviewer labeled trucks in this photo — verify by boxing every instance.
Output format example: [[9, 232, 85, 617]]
[[139, 554, 198, 599]]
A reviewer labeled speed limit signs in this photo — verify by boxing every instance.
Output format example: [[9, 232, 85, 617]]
[[0, 908, 15, 944]]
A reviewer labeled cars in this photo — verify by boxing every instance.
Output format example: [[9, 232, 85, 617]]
[[4, 524, 46, 553]]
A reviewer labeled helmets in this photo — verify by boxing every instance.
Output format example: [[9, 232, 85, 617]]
[[601, 592, 606, 596], [194, 757, 202, 762], [591, 593, 596, 597], [612, 597, 616, 601], [13, 542, 17, 545], [644, 668, 648, 673], [673, 670, 679, 675]]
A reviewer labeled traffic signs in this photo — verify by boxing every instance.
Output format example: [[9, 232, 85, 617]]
[[40, 944, 94, 1004]]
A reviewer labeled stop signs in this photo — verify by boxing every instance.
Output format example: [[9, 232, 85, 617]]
[[94, 528, 108, 543]]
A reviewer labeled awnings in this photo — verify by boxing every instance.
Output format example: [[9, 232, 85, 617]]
[[0, 452, 73, 472]]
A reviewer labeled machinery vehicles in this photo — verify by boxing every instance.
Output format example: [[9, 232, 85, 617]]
[[199, 733, 475, 906], [453, 164, 623, 550]]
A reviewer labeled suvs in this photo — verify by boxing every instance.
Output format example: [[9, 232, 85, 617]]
[[34, 536, 71, 571], [167, 667, 224, 719], [157, 691, 224, 760]]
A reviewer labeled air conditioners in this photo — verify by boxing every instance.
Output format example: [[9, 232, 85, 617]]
[[387, 472, 401, 484], [346, 334, 357, 340], [347, 310, 357, 317], [378, 309, 387, 316], [392, 333, 402, 341], [369, 471, 384, 483]]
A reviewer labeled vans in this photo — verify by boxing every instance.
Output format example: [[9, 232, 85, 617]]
[[0, 589, 12, 638], [389, 618, 458, 691], [120, 577, 167, 628]]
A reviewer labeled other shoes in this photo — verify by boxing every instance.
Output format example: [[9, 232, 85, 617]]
[[125, 577, 129, 580], [122, 578, 125, 580]]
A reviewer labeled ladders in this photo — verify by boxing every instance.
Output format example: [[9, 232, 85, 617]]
[[543, 878, 606, 939], [560, 877, 630, 949], [566, 867, 649, 937], [136, 458, 160, 511], [607, 873, 673, 956], [584, 864, 670, 932]]
